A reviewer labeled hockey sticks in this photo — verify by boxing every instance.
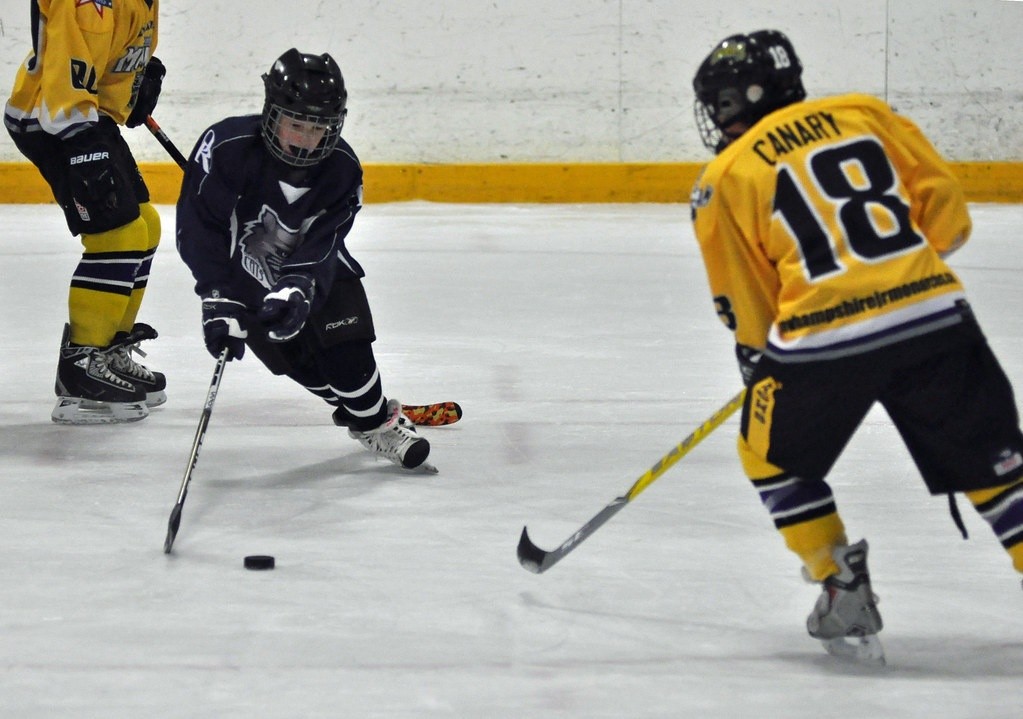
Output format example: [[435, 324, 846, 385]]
[[515, 384, 748, 575], [141, 114, 463, 554]]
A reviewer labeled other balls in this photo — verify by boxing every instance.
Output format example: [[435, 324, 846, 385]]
[[243, 555, 276, 571]]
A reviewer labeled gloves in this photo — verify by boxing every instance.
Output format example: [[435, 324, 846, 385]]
[[125, 56, 166, 128], [64, 121, 140, 217], [202, 298, 247, 362], [736, 344, 763, 386], [257, 272, 315, 343]]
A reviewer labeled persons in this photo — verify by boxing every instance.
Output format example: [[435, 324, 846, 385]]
[[690, 29, 1023, 668], [176, 48, 439, 473], [4, 0, 167, 423]]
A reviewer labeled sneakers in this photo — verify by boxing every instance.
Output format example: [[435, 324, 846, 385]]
[[332, 396, 439, 474], [107, 323, 166, 407], [51, 323, 149, 426], [802, 538, 886, 670]]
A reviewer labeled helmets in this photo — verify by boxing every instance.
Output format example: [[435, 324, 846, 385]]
[[260, 48, 346, 167], [691, 30, 807, 155]]
